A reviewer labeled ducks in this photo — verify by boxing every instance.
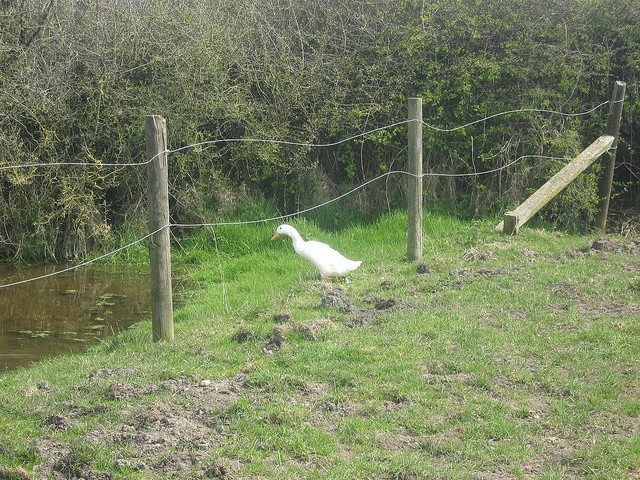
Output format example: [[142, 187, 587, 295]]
[[271, 224, 363, 282]]
